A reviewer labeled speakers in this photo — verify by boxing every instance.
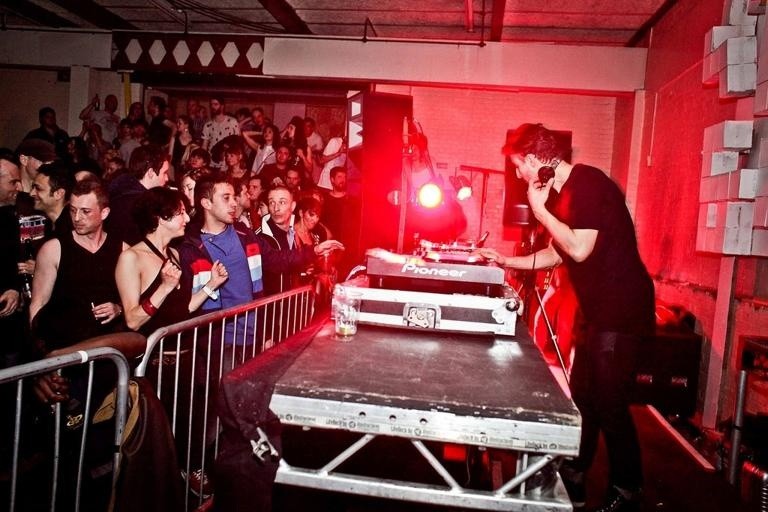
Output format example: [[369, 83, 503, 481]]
[[502, 129, 572, 226]]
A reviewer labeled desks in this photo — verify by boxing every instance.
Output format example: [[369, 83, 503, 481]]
[[268, 317, 583, 512]]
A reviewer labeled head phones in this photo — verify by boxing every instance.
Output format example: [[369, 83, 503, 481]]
[[538, 155, 563, 183]]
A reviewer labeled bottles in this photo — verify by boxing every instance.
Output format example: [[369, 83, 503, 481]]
[[20, 238, 34, 304]]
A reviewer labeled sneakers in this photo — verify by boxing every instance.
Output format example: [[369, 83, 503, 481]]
[[180, 468, 211, 499], [595, 485, 651, 512], [563, 479, 588, 506]]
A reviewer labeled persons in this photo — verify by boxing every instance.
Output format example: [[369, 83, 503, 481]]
[[2, 96, 362, 498], [467, 122, 655, 512]]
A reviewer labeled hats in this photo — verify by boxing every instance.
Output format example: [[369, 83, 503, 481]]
[[15, 139, 60, 162]]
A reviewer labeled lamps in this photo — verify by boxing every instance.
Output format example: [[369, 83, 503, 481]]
[[387, 144, 443, 209], [449, 176, 473, 200]]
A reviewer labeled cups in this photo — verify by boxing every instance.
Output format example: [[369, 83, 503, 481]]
[[335, 288, 362, 335]]
